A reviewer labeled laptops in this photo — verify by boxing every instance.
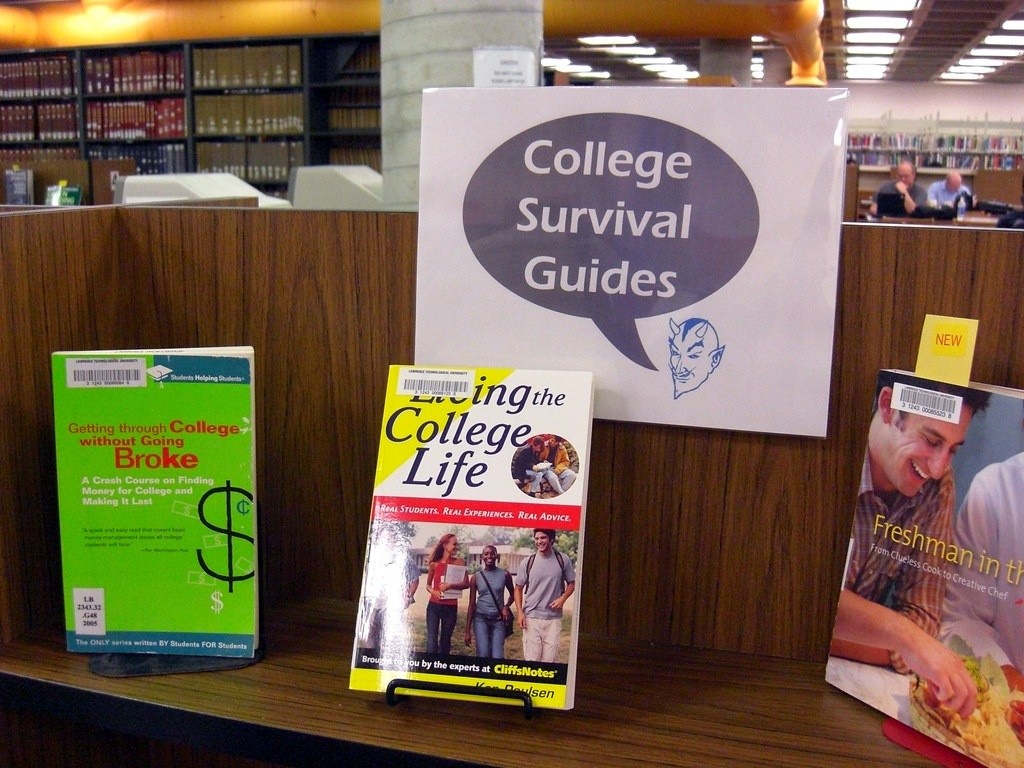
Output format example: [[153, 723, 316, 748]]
[[876, 192, 905, 219]]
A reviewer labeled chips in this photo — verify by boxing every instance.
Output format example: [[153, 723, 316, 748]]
[[912, 684, 1024, 767]]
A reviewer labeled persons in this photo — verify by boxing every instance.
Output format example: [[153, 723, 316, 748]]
[[404, 556, 422, 609], [942, 450, 1024, 676], [868, 160, 928, 218], [927, 172, 970, 214], [514, 528, 575, 663], [513, 436, 576, 498], [830, 371, 993, 720], [424, 533, 470, 655], [464, 545, 515, 659]]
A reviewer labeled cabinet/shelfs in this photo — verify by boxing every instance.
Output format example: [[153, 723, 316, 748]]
[[0, 31, 383, 191], [847, 129, 1024, 175]]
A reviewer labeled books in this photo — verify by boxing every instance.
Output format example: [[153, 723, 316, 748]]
[[45, 184, 83, 206], [327, 43, 382, 174], [348, 365, 595, 710], [847, 132, 1024, 170], [51, 345, 259, 658], [6, 169, 34, 205], [0, 55, 81, 161], [193, 45, 304, 182], [81, 51, 187, 176], [440, 564, 466, 599], [825, 369, 1024, 768]]
